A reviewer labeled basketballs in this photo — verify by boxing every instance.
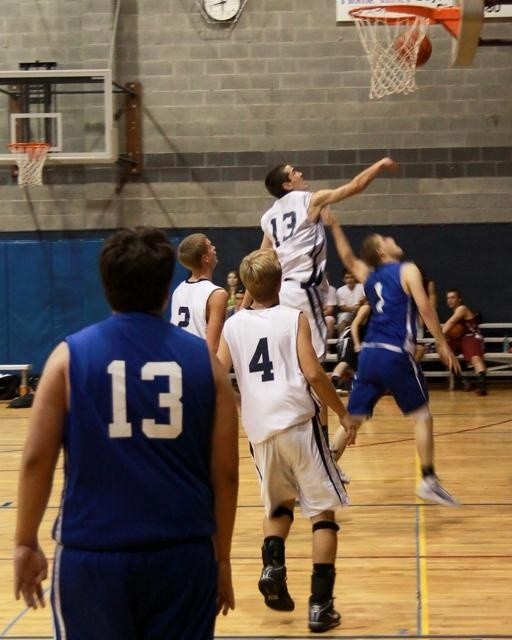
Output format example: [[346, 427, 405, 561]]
[[447, 323, 464, 338], [394, 33, 431, 68]]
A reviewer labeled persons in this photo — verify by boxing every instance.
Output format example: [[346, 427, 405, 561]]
[[11, 225, 239, 640], [239, 155, 399, 484], [327, 267, 488, 398], [225, 289, 245, 319], [168, 232, 229, 351], [223, 270, 241, 306], [217, 247, 357, 634], [331, 232, 464, 507]]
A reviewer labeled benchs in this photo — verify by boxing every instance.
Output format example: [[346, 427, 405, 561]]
[[0, 363, 33, 397], [228, 321, 511, 392]]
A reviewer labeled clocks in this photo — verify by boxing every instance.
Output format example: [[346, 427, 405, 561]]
[[202, 0, 242, 25]]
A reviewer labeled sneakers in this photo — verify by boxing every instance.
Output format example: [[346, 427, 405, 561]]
[[257, 564, 295, 612], [327, 442, 350, 485], [415, 475, 459, 508], [307, 596, 343, 631], [449, 371, 489, 395], [330, 373, 355, 395]]
